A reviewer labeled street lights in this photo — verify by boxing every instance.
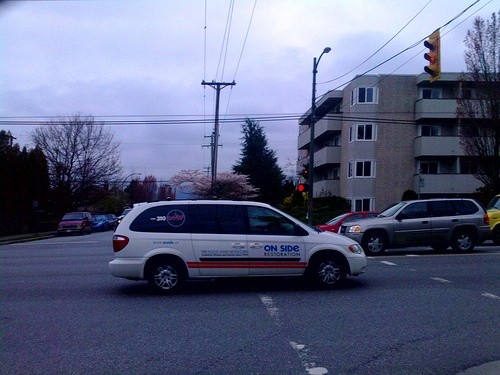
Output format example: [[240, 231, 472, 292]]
[[308, 47, 331, 224]]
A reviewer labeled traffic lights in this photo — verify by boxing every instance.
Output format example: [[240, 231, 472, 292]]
[[423, 29, 441, 82], [298, 164, 310, 192]]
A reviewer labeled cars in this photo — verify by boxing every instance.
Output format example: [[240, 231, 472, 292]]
[[109, 199, 368, 295], [313, 211, 380, 233], [106, 214, 117, 230], [338, 198, 490, 256], [93, 214, 109, 232], [116, 208, 132, 229], [487, 194, 500, 246]]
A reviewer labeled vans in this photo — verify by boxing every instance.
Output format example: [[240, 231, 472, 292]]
[[58, 212, 93, 235]]
